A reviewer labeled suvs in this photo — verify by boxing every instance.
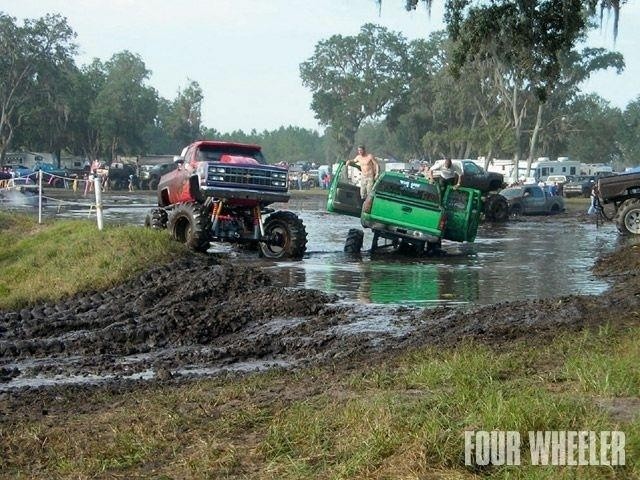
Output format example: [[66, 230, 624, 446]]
[[275, 156, 419, 191], [0, 157, 178, 209], [432, 154, 640, 237], [324, 158, 484, 261], [143, 139, 310, 263]]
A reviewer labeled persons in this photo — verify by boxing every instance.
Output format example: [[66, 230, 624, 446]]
[[101, 171, 111, 194], [542, 182, 550, 193], [127, 175, 135, 192], [346, 145, 380, 202], [586, 188, 599, 216], [428, 158, 463, 200], [288, 172, 330, 190], [549, 182, 558, 197], [417, 160, 429, 178]]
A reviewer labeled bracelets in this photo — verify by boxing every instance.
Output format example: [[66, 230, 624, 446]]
[[456, 183, 461, 186]]
